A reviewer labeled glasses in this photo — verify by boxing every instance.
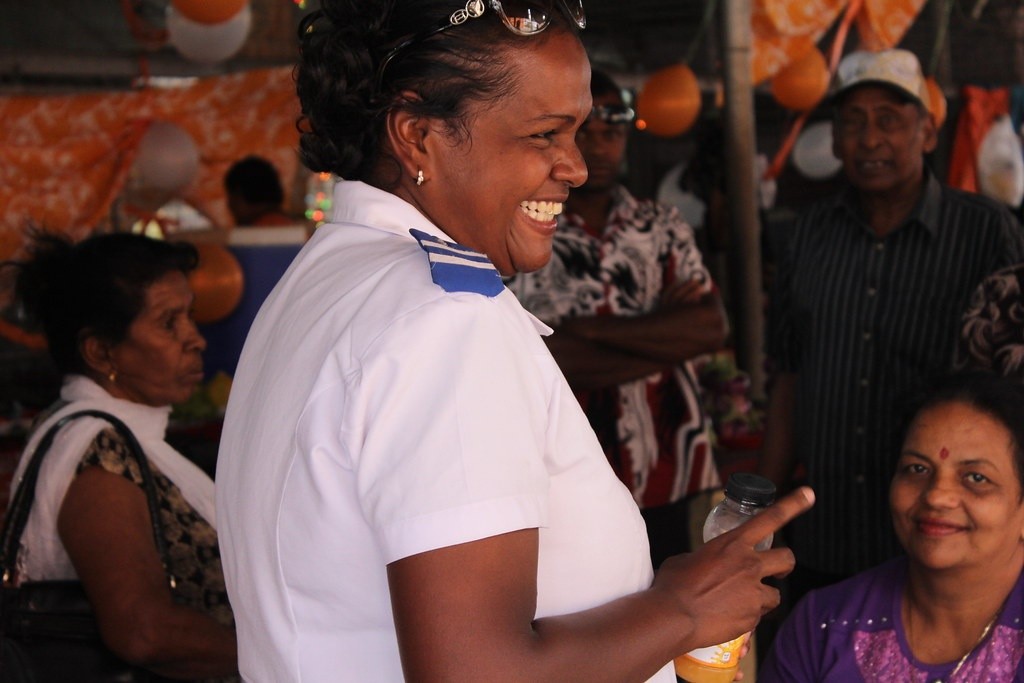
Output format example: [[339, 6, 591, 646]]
[[370, 0, 586, 101], [583, 104, 635, 127]]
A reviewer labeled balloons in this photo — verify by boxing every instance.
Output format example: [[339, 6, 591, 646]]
[[637, 65, 701, 132], [163, 0, 255, 60], [765, 40, 830, 106]]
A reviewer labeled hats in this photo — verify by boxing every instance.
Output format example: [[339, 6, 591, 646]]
[[825, 49, 931, 115]]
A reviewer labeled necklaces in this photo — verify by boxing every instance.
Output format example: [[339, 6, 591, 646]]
[[902, 582, 997, 683]]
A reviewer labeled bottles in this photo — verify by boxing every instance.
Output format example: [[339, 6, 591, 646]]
[[674, 470, 779, 683]]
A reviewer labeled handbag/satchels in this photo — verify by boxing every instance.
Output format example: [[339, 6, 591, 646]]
[[0, 409, 179, 683]]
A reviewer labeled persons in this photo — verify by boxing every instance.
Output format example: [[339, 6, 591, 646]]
[[0, 0, 1024, 683]]
